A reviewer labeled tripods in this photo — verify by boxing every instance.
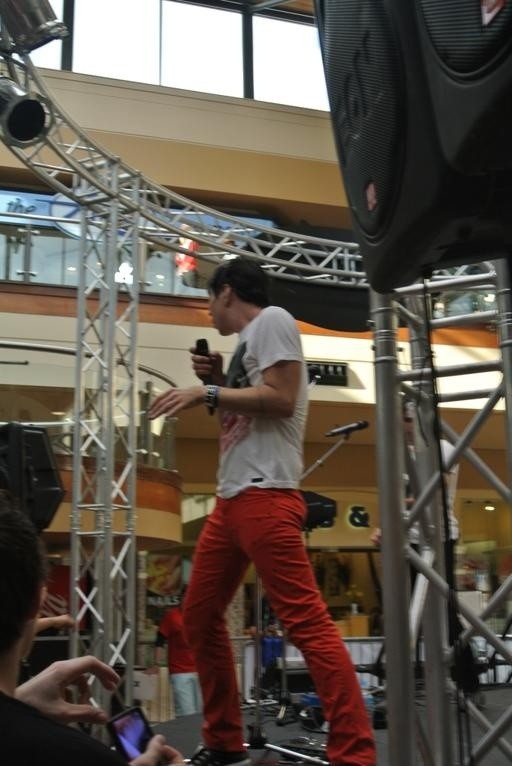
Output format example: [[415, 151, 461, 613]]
[[242, 617, 315, 724], [242, 439, 348, 766]]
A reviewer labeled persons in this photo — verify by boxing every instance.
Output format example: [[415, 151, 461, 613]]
[[366, 393, 486, 732], [140, 256, 379, 766], [173, 221, 202, 294], [243, 618, 284, 638], [0, 500, 189, 765], [34, 614, 77, 636], [155, 584, 204, 723]]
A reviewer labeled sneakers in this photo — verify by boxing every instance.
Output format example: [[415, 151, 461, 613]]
[[180, 744, 252, 766]]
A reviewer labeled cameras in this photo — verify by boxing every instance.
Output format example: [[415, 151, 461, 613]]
[[106, 706, 167, 766]]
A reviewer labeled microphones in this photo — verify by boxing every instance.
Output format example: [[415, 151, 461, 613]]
[[326, 420, 368, 437], [197, 339, 215, 415]]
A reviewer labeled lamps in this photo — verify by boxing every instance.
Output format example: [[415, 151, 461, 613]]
[[0, 0, 70, 59], [0, 76, 56, 148]]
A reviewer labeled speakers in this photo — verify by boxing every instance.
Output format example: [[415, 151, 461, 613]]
[[311, 0, 512, 292]]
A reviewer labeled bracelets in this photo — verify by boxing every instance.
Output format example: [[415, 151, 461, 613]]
[[204, 383, 219, 409]]
